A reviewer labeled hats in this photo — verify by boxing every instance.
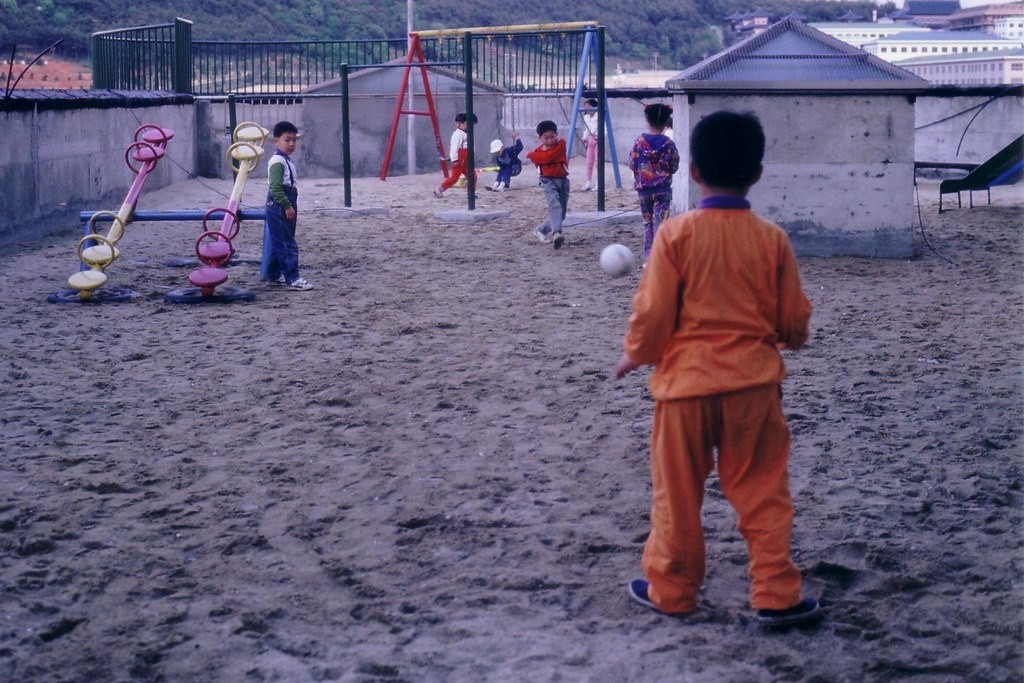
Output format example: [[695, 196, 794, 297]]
[[490, 140, 503, 153]]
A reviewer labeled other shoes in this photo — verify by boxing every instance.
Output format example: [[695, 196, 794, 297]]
[[591, 187, 598, 191], [466, 194, 480, 199], [434, 186, 442, 198], [582, 185, 590, 191], [485, 185, 504, 192]]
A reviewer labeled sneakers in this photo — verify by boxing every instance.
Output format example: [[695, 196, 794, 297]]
[[628, 578, 662, 611], [265, 275, 287, 286], [286, 277, 314, 291], [756, 594, 819, 623], [533, 227, 551, 244], [553, 232, 565, 250]]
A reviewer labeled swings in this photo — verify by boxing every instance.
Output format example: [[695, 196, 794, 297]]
[[433, 37, 462, 161], [540, 33, 598, 150], [487, 39, 522, 176]]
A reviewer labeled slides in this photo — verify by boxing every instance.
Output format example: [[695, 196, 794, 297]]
[[939, 132, 1024, 194]]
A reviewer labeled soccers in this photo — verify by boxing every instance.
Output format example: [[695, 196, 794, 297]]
[[600, 243, 635, 277]]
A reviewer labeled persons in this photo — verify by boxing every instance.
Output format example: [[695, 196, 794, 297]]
[[628, 103, 680, 268], [581, 99, 607, 192], [266, 120, 314, 290], [484, 131, 524, 192], [615, 109, 820, 624], [527, 120, 570, 250], [434, 112, 479, 198]]
